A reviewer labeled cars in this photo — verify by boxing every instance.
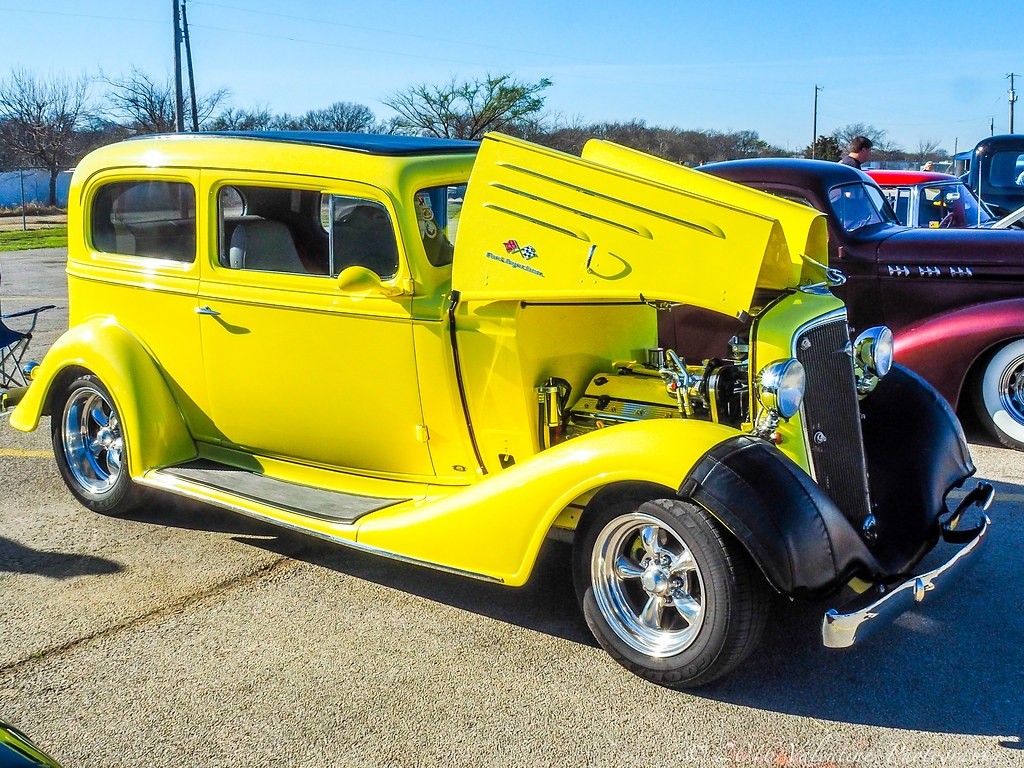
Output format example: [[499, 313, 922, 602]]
[[668, 155, 1024, 452], [951, 135, 1024, 230], [857, 169, 1014, 231], [13, 128, 996, 694]]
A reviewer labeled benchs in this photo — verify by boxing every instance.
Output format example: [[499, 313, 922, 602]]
[[103, 211, 326, 276]]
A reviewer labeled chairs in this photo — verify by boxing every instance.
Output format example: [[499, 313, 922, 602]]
[[230, 220, 310, 274], [334, 206, 400, 274], [0, 273, 57, 389]]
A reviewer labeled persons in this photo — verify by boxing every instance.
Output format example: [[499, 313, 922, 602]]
[[830, 136, 873, 229], [923, 161, 933, 172]]
